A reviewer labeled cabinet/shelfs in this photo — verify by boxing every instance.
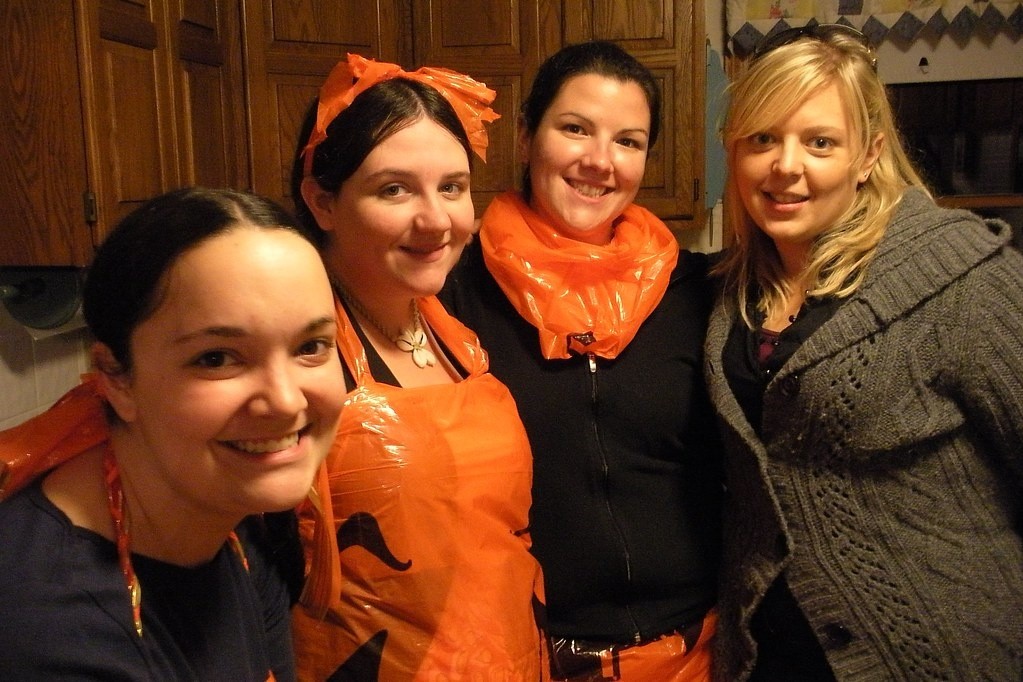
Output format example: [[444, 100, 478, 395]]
[[0, 0, 709, 269]]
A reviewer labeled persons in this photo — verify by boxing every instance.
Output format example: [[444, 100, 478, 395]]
[[438, 42, 737, 682], [0, 187, 346, 682], [289, 53, 550, 682], [704, 26, 1022, 682]]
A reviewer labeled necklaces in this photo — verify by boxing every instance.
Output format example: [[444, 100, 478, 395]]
[[119, 429, 179, 563], [331, 276, 438, 369]]
